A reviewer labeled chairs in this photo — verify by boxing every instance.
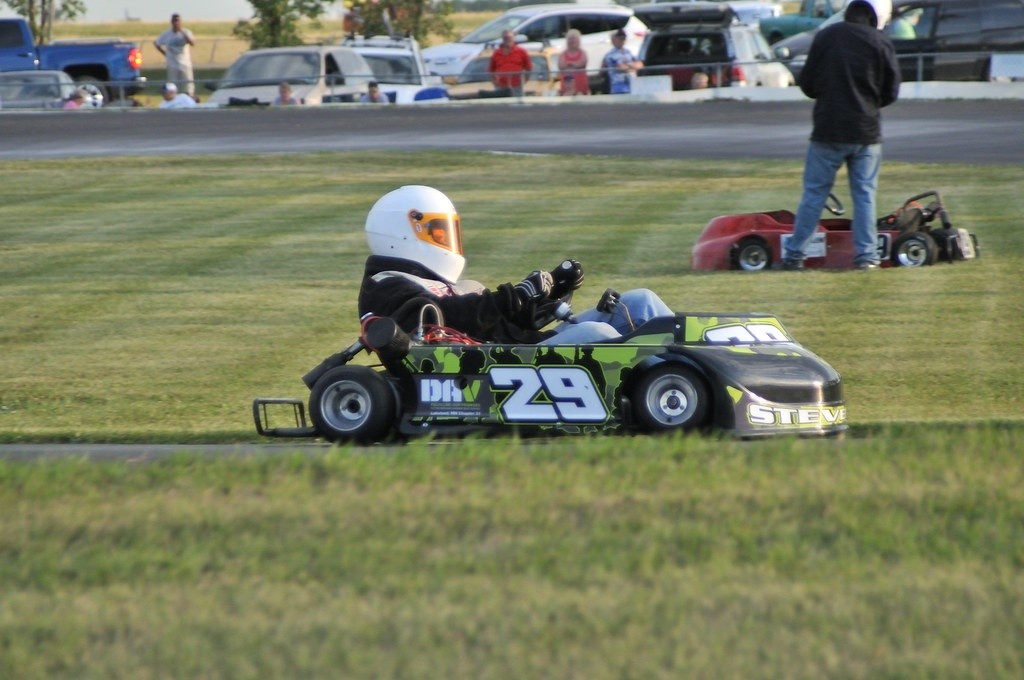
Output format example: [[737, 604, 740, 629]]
[[676, 40, 691, 53]]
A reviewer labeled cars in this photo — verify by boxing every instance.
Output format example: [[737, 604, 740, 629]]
[[209, 34, 442, 104], [424, 2, 652, 84], [0, 70, 87, 111], [625, 3, 794, 88], [755, 1, 855, 45]]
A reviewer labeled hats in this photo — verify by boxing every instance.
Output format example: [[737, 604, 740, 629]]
[[162, 83, 176, 91]]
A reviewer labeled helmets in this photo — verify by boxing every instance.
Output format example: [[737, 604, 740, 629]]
[[846, 1, 893, 31], [363, 184, 465, 286]]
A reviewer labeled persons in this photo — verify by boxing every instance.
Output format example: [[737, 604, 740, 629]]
[[154, 14, 195, 96], [160, 82, 195, 108], [777, 0, 901, 271], [691, 73, 708, 88], [63, 91, 85, 109], [357, 184, 675, 343], [559, 28, 589, 96], [361, 81, 389, 102], [599, 32, 643, 93], [274, 82, 301, 104], [488, 30, 533, 97], [884, 2, 923, 39]]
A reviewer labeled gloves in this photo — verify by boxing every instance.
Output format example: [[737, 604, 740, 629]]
[[554, 259, 584, 291], [513, 270, 553, 300]]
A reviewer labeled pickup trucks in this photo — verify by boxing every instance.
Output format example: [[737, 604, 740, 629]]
[[0, 17, 146, 110]]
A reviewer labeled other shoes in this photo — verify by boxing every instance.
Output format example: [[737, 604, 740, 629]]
[[856, 263, 877, 271], [782, 259, 804, 271]]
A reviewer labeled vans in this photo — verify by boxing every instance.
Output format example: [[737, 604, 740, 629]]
[[767, 0, 1024, 83]]
